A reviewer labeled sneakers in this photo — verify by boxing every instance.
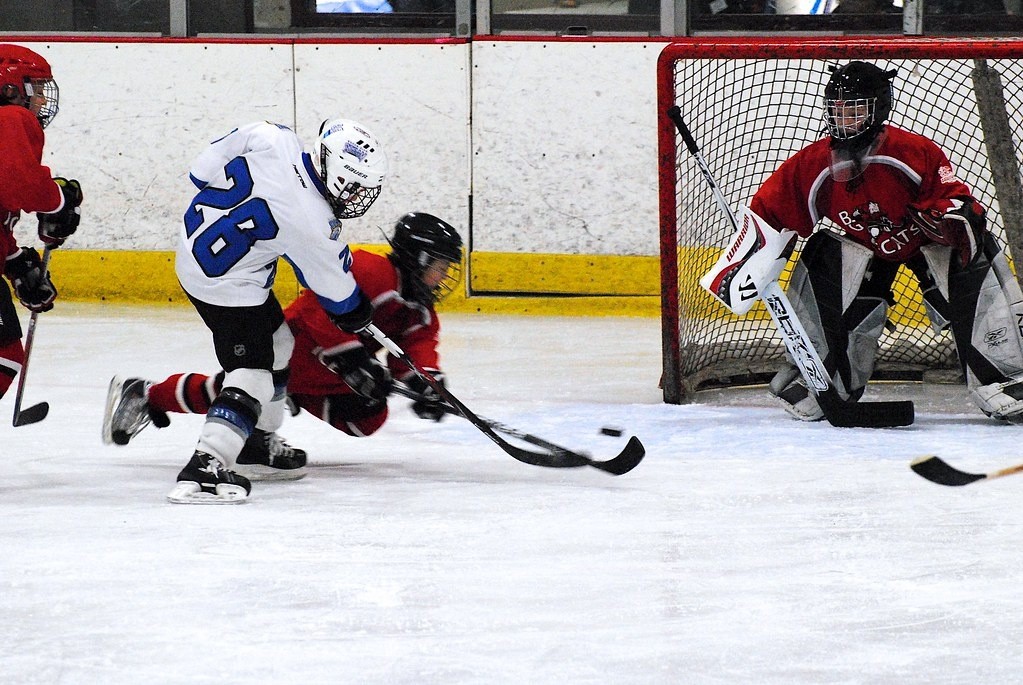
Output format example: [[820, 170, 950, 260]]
[[101, 374, 171, 445], [234, 427, 309, 481], [166, 449, 251, 504]]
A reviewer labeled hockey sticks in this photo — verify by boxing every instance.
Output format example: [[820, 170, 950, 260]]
[[391, 383, 645, 477], [668, 104, 916, 428], [366, 324, 592, 468], [910, 453, 1023, 487], [12, 243, 49, 428]]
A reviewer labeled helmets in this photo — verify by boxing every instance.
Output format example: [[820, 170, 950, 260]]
[[0, 43, 59, 128], [823, 61, 898, 152], [388, 212, 464, 305], [312, 118, 387, 219]]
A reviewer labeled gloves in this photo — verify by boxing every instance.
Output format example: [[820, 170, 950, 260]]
[[324, 287, 376, 334], [3, 246, 57, 313], [401, 366, 447, 422], [318, 341, 396, 402], [36, 177, 84, 250]]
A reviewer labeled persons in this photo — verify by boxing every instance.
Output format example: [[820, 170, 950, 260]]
[[101, 210, 462, 450], [167, 117, 388, 506], [700, 60, 1023, 427], [0, 43, 85, 399]]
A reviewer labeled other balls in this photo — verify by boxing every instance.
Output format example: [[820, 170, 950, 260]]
[[602, 428, 622, 438]]
